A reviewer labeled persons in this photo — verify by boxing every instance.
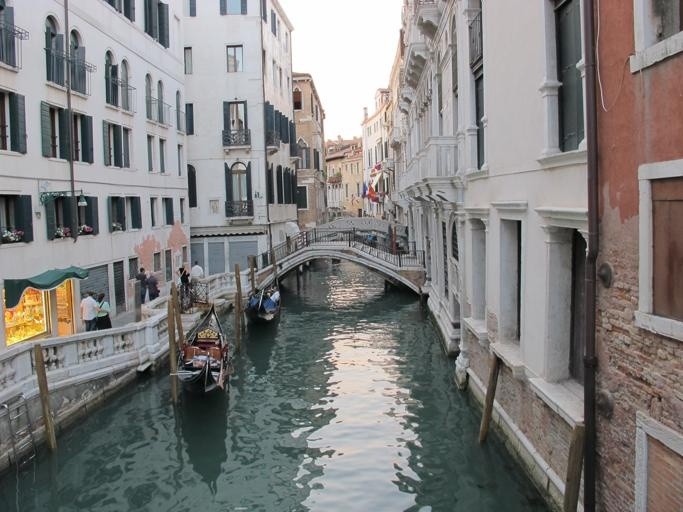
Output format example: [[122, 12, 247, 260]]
[[175, 263, 190, 285], [191, 261, 203, 279], [136, 267, 160, 304], [80, 291, 112, 332]]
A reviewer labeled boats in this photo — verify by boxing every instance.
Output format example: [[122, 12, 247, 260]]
[[176, 298, 235, 395], [240, 283, 287, 325]]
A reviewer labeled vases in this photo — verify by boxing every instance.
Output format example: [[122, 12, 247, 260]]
[[2, 237, 11, 243], [79, 230, 87, 235]]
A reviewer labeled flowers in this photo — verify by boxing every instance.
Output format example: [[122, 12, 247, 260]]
[[54, 225, 71, 240], [76, 223, 94, 236], [1, 228, 25, 243]]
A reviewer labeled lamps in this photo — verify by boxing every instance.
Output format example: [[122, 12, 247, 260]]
[[38, 188, 87, 209]]
